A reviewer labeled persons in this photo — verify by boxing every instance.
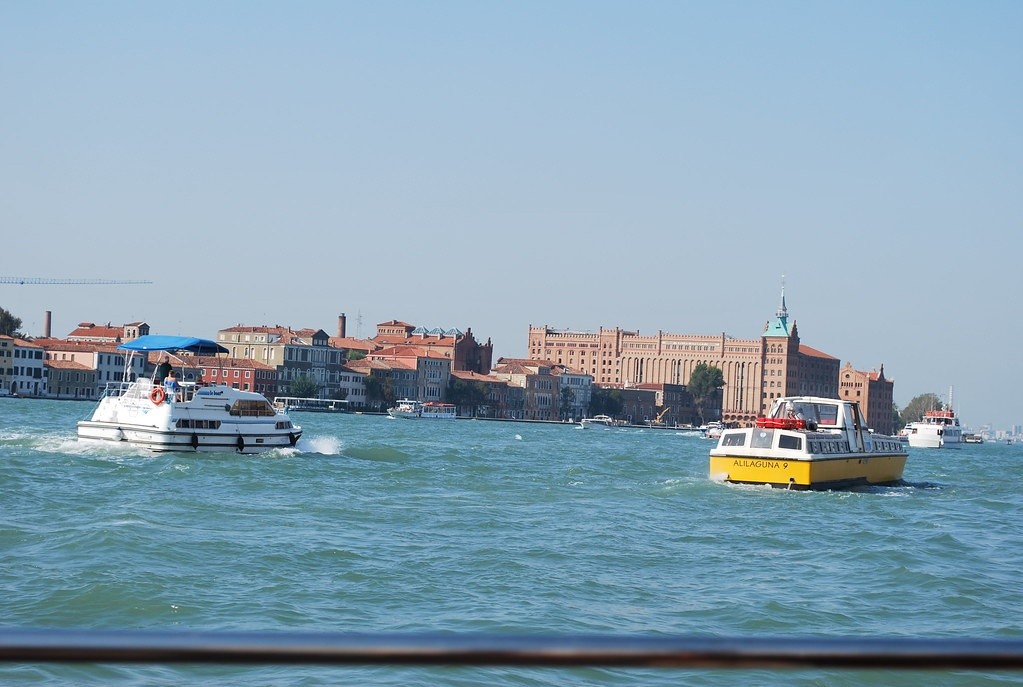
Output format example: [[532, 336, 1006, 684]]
[[195, 374, 207, 390], [164, 370, 180, 401], [160, 356, 172, 382], [788, 408, 804, 420]]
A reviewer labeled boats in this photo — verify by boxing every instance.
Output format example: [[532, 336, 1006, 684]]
[[387, 398, 457, 420], [698, 397, 909, 491], [274, 396, 349, 412], [77, 335, 302, 452], [901, 386, 983, 448], [579, 414, 612, 430]]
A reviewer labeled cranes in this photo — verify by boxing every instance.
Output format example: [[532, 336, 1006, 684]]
[[0, 277, 153, 284]]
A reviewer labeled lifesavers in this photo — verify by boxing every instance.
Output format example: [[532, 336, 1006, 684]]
[[149, 387, 166, 405]]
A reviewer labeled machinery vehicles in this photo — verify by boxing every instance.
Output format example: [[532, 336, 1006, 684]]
[[652, 407, 671, 426]]
